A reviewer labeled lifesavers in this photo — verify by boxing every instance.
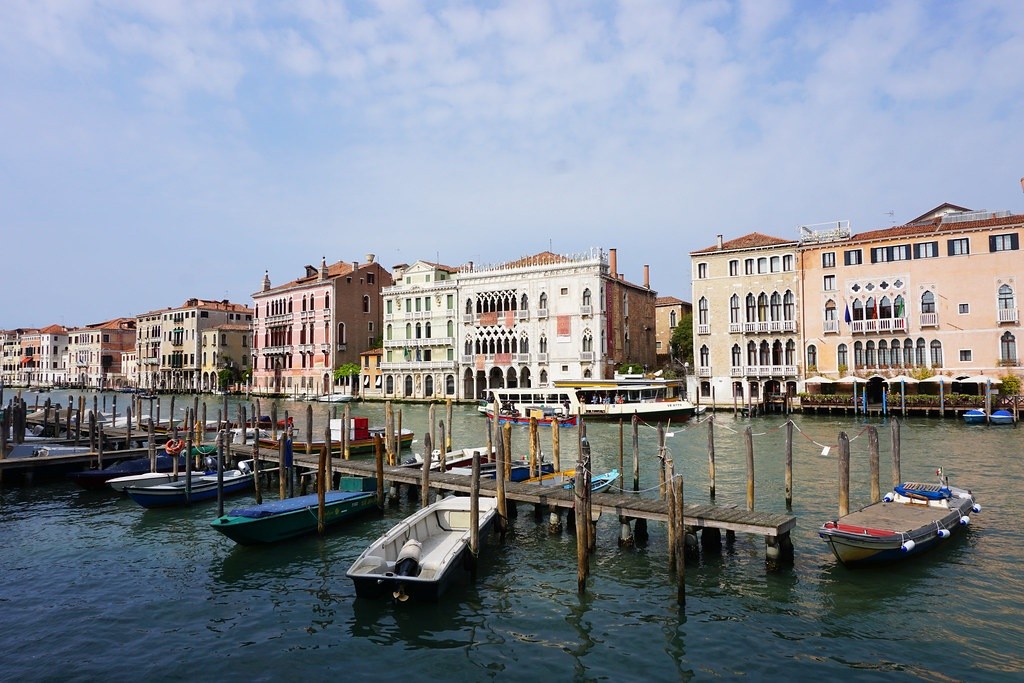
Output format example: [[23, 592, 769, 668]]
[[165, 438, 185, 455]]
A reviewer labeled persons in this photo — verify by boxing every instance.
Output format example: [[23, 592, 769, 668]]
[[593, 392, 611, 404], [288, 422, 293, 443], [485, 394, 495, 404], [617, 396, 623, 404], [563, 399, 570, 418], [502, 400, 515, 411], [580, 396, 586, 404]]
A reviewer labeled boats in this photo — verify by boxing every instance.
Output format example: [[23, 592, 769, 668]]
[[208, 477, 391, 547], [122, 461, 263, 509], [564, 469, 619, 493], [420, 446, 497, 472], [0, 441, 167, 476], [817, 465, 982, 563], [485, 399, 583, 428], [989, 410, 1014, 424], [74, 451, 197, 488], [445, 452, 555, 482], [695, 405, 707, 414], [478, 379, 695, 424], [520, 468, 577, 487], [258, 426, 414, 457], [963, 409, 987, 424], [104, 471, 206, 491], [398, 452, 425, 468], [317, 394, 353, 401], [0, 381, 314, 454], [345, 495, 499, 600]]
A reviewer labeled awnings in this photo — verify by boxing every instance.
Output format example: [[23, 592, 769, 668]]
[[22, 357, 33, 363]]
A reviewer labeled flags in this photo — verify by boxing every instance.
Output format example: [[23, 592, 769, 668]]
[[936, 467, 942, 476], [898, 299, 905, 328], [873, 297, 878, 328], [845, 303, 851, 324]]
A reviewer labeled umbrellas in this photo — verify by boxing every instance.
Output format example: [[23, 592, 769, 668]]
[[961, 370, 1004, 383], [921, 371, 959, 384], [882, 373, 919, 384], [833, 375, 870, 383], [802, 375, 832, 383]]
